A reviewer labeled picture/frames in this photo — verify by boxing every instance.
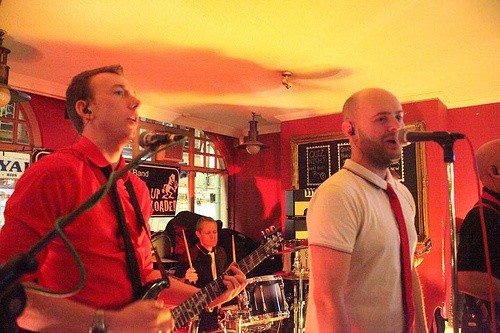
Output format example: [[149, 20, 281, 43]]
[[289, 119, 426, 244]]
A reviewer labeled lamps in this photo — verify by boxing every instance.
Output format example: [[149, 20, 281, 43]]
[[0, 29, 31, 105], [238, 112, 269, 154]]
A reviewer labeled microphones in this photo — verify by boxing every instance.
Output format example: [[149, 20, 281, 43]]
[[394, 126, 466, 147], [137, 133, 184, 149]]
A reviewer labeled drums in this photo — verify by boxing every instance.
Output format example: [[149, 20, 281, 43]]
[[217, 304, 247, 333], [292, 247, 310, 279], [236, 273, 290, 327]]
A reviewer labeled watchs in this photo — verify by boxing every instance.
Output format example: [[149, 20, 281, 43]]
[[89, 310, 106, 333]]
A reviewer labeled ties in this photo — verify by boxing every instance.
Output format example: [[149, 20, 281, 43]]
[[208, 252, 222, 313], [385, 183, 416, 333], [100, 164, 143, 299]]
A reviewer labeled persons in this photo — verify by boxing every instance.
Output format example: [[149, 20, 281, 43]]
[[305, 88, 428, 333], [176, 216, 228, 288], [0, 64, 249, 333], [456, 139, 500, 333]]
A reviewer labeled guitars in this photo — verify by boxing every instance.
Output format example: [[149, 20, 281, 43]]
[[138, 225, 285, 333]]
